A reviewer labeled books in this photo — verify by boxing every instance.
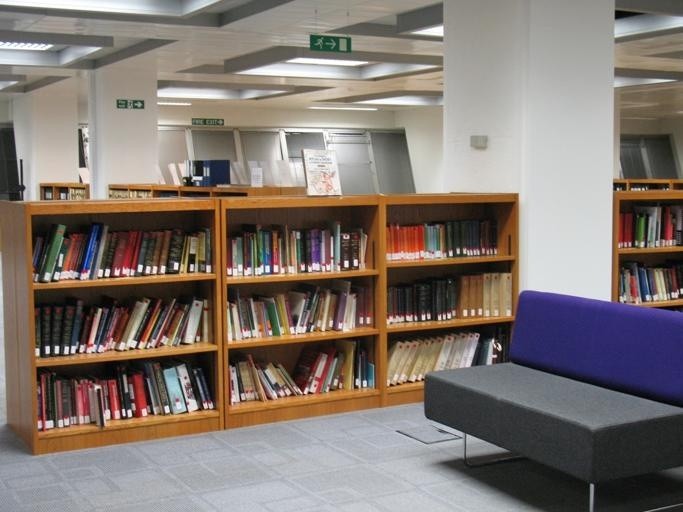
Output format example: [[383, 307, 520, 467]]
[[387, 326, 506, 388], [616, 200, 683, 306], [228, 338, 374, 405], [36, 362, 213, 428], [182, 159, 232, 189], [32, 224, 211, 283], [387, 274, 511, 325], [384, 217, 497, 260], [215, 183, 251, 190], [225, 221, 369, 276], [227, 280, 374, 341], [33, 294, 208, 358], [300, 148, 342, 198], [41, 186, 151, 201]]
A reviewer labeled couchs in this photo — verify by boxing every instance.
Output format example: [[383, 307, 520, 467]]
[[423, 290, 683, 511]]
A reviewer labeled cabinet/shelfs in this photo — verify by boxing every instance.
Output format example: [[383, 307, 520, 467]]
[[4, 197, 224, 455], [384, 195, 520, 407], [110, 182, 309, 198], [224, 193, 383, 428], [38, 182, 88, 200], [610, 178, 683, 307]]
